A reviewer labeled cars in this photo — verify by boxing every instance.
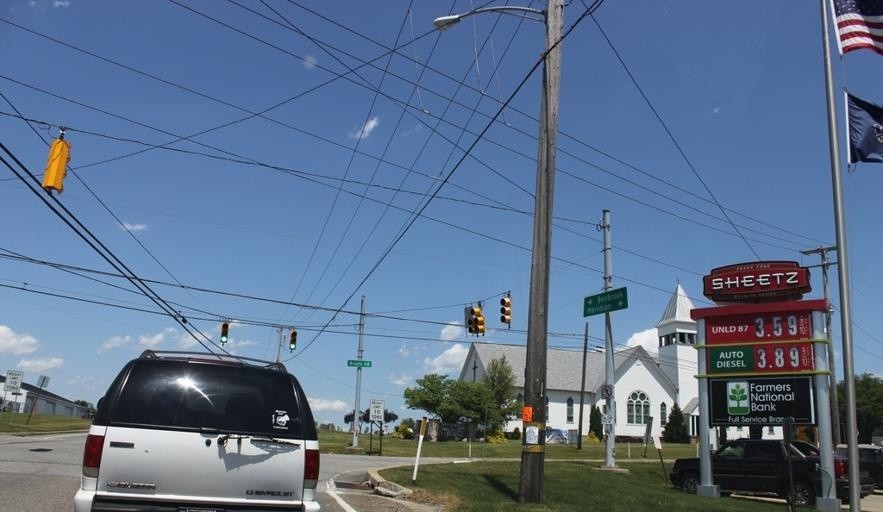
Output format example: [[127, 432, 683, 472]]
[[669, 439, 883, 506], [73, 349, 321, 512]]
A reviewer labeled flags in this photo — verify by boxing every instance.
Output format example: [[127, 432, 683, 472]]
[[844, 91, 883, 164], [829, 0, 883, 57]]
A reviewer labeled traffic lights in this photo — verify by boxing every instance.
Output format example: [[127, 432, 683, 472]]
[[500, 296, 512, 323], [468, 306, 485, 334], [220, 322, 228, 343], [289, 331, 297, 350], [42, 139, 72, 193]]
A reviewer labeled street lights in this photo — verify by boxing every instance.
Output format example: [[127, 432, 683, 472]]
[[433, 5, 563, 504]]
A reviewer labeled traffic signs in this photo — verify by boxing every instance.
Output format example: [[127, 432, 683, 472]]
[[583, 286, 628, 317]]
